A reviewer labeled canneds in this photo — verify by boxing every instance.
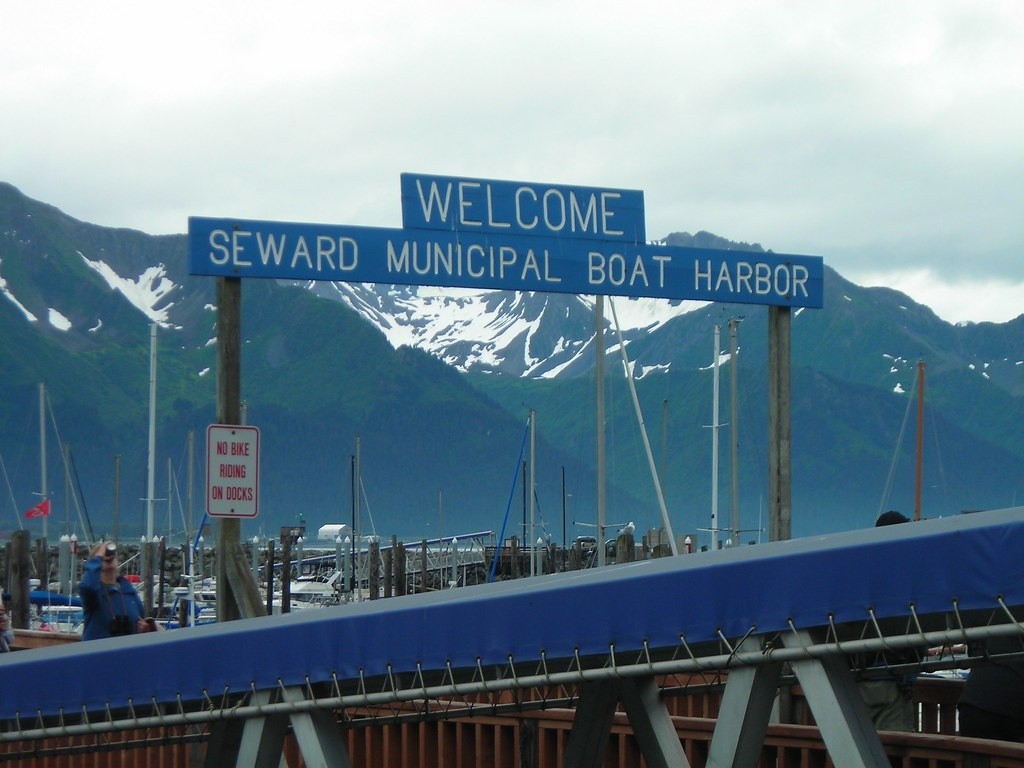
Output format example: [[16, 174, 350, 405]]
[[105, 542, 116, 563]]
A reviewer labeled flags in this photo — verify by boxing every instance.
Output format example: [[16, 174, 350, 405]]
[[24, 500, 50, 519]]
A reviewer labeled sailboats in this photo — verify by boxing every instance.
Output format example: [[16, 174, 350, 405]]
[[0, 293, 952, 651]]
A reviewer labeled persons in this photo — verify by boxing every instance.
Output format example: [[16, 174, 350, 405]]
[[78, 539, 157, 640], [956, 636, 1024, 742], [848, 510, 928, 732], [0, 604, 13, 654]]
